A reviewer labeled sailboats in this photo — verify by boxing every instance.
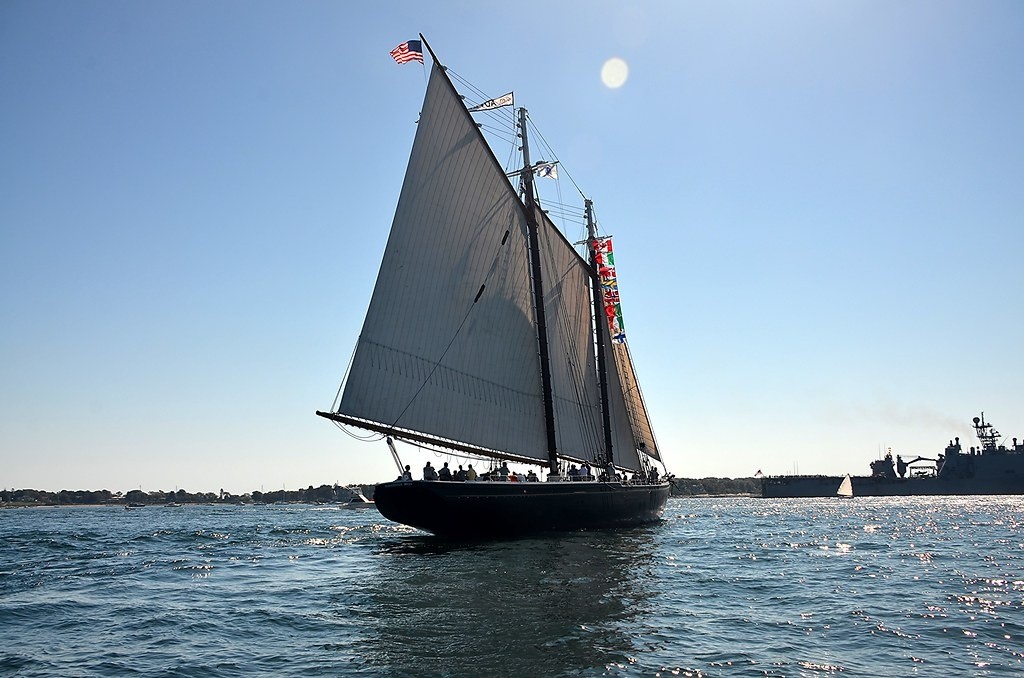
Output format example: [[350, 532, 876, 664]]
[[314, 30, 669, 539]]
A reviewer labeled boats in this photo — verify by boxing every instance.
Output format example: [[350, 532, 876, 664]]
[[847, 412, 1024, 495], [761, 475, 846, 498]]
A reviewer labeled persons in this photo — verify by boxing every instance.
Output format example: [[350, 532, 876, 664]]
[[401, 460, 659, 485]]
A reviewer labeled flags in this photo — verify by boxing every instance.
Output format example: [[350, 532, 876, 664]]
[[537, 163, 559, 180], [592, 239, 627, 344], [389, 40, 424, 64]]
